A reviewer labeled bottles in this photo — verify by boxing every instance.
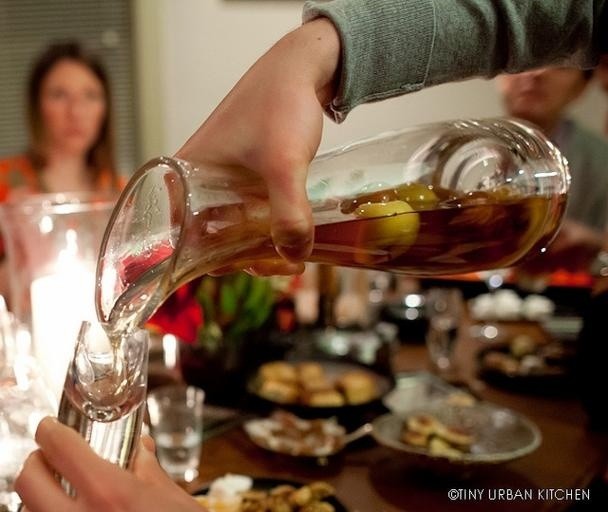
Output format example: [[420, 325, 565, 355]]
[[95, 115, 573, 336]]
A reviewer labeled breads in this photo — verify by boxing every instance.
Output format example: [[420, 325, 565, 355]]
[[256, 362, 374, 407]]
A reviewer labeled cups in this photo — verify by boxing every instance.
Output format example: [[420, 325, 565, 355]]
[[145, 385, 204, 484], [0, 191, 150, 501]]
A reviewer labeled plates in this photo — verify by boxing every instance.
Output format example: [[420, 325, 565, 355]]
[[192, 337, 574, 512]]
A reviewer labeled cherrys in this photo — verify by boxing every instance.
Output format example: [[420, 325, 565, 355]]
[[339, 183, 539, 267]]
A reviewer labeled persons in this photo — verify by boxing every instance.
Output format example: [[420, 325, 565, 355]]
[[15, 1, 608, 512], [497, 68, 608, 257], [0, 43, 128, 312]]
